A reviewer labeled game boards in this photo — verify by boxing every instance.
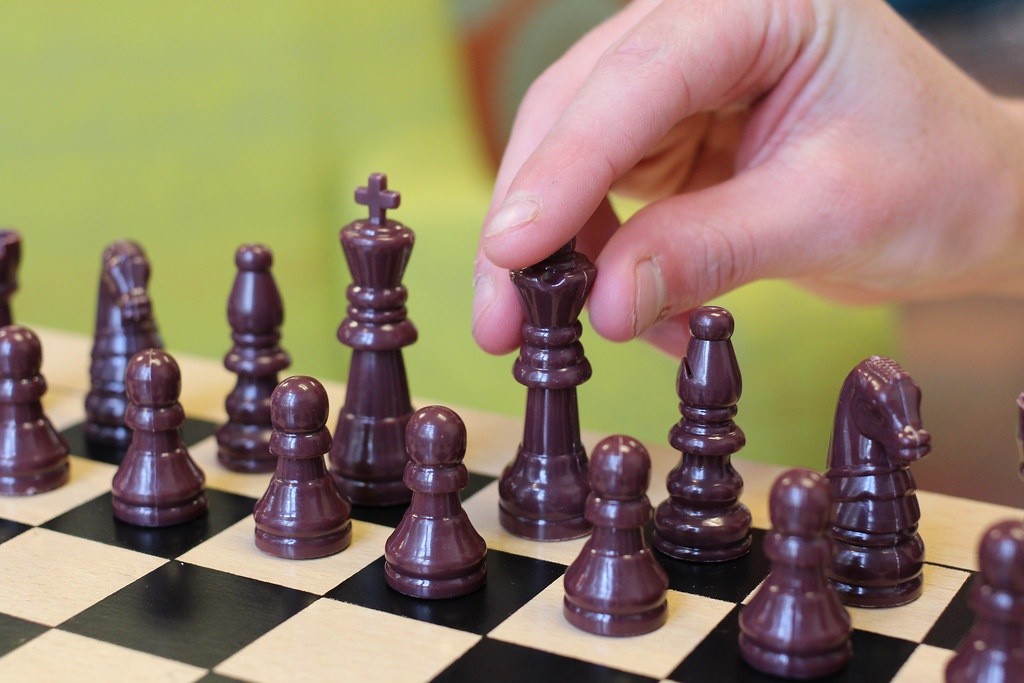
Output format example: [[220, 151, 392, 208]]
[[0, 322, 1022, 683]]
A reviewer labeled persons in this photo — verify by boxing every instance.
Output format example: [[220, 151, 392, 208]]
[[472, 0, 1024, 510]]
